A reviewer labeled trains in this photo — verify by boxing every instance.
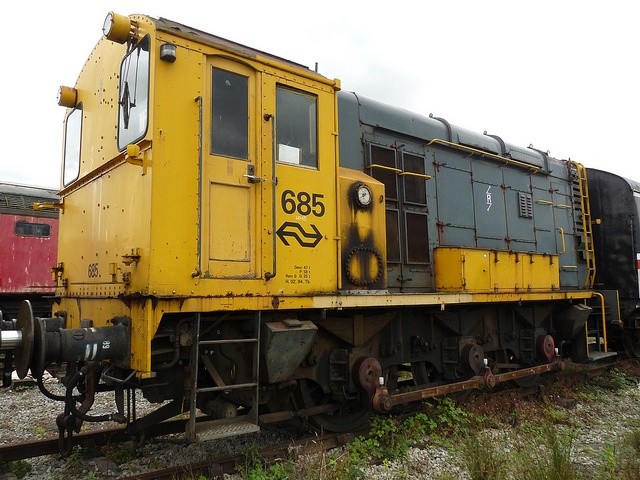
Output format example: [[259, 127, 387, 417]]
[[1, 11, 640, 447], [1, 183, 60, 391]]
